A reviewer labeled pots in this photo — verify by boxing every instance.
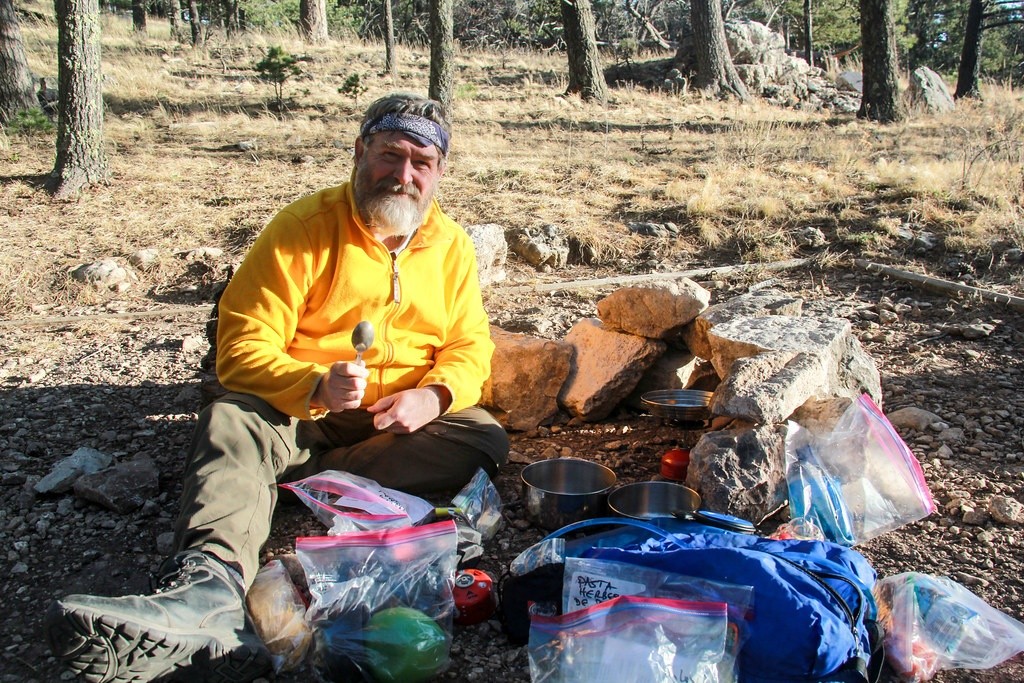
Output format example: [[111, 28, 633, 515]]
[[608, 478, 701, 521], [640, 388, 714, 421], [520, 456, 619, 531]]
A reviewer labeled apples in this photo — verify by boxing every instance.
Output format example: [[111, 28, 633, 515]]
[[362, 606, 449, 683]]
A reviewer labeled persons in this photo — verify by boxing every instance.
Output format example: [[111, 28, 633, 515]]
[[44, 94, 509, 683]]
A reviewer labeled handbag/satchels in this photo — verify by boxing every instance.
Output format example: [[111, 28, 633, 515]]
[[498, 515, 884, 683]]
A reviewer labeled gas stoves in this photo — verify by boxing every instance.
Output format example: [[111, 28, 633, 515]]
[[651, 419, 712, 447]]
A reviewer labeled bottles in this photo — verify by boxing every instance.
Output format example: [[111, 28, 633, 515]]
[[787, 444, 853, 548]]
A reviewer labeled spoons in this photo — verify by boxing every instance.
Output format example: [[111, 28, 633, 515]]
[[351, 321, 373, 366]]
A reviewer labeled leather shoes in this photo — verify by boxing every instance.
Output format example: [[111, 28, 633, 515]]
[[44, 555, 271, 683]]
